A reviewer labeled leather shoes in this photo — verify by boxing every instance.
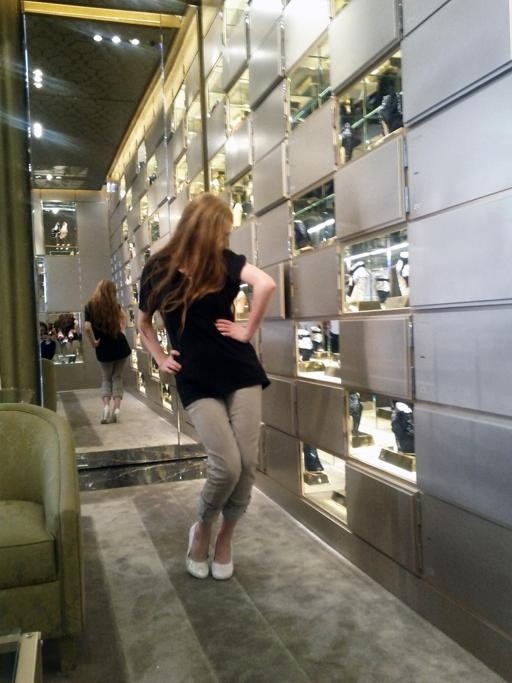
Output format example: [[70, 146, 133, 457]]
[[185, 521, 210, 579], [212, 528, 234, 580], [303, 444, 324, 472]]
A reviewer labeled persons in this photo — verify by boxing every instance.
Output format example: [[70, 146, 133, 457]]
[[84, 279, 131, 424], [137, 193, 276, 581], [40, 322, 57, 359], [55, 312, 80, 355]]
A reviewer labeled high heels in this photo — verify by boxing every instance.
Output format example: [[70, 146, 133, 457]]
[[391, 409, 413, 453], [100, 405, 110, 423], [350, 392, 362, 435], [110, 408, 120, 424]]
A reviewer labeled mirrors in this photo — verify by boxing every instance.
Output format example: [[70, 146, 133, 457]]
[[20, 9, 229, 481]]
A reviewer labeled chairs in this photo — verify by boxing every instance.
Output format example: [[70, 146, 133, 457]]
[[0, 400, 91, 642]]
